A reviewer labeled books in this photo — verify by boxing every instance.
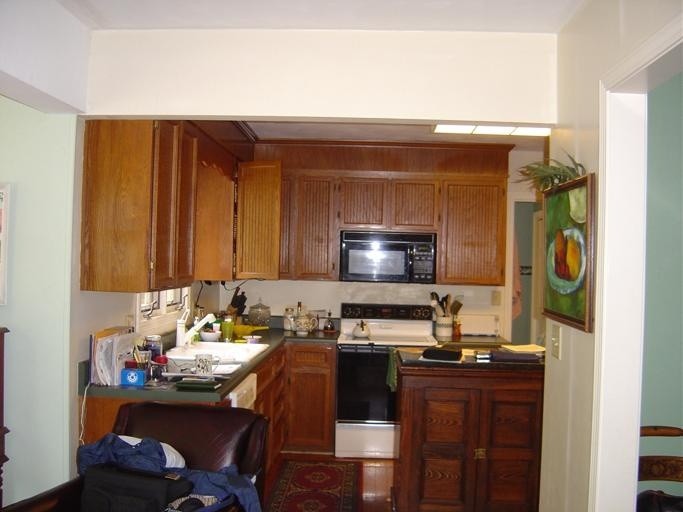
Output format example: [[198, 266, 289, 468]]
[[176, 381, 221, 390], [489, 351, 538, 363], [418, 355, 465, 365], [161, 371, 231, 380], [182, 376, 215, 383], [422, 349, 462, 361], [500, 344, 545, 353], [190, 364, 241, 375]]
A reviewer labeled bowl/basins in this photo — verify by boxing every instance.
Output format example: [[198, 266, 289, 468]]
[[199, 331, 220, 341]]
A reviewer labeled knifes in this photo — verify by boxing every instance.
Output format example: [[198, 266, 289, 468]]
[[230, 287, 246, 316]]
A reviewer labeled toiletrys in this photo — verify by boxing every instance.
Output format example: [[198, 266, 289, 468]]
[[222, 319, 234, 342]]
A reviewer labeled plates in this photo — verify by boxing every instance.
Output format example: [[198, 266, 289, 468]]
[[418, 353, 463, 363], [547, 228, 587, 294]]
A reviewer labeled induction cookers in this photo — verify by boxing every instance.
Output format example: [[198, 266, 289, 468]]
[[336, 332, 438, 345]]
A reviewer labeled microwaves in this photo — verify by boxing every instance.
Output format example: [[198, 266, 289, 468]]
[[339, 231, 435, 285]]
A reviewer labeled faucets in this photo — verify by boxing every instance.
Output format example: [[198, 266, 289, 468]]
[[186, 314, 217, 340]]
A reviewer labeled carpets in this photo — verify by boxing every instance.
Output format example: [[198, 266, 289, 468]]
[[261, 453, 364, 512]]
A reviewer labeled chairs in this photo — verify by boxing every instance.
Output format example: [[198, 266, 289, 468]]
[[1, 398, 267, 510]]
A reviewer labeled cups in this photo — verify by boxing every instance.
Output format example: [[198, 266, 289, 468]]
[[436, 316, 452, 341], [220, 321, 234, 340], [195, 354, 220, 378]]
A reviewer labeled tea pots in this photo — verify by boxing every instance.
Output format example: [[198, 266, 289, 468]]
[[352, 320, 370, 337]]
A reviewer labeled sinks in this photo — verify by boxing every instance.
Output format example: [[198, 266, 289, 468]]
[[167, 341, 270, 364]]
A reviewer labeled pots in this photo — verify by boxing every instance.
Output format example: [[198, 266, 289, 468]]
[[233, 325, 268, 337]]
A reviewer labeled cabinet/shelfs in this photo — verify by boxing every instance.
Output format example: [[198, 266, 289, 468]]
[[332, 136, 441, 232], [285, 335, 340, 464], [254, 354, 273, 509], [252, 139, 334, 283], [269, 337, 287, 491], [190, 120, 282, 284], [432, 137, 517, 287], [385, 363, 548, 511], [78, 118, 194, 295]]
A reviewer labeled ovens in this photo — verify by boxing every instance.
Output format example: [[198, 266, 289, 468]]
[[335, 344, 404, 460]]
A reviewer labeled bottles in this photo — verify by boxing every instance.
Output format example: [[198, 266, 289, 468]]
[[248, 298, 270, 331], [193, 304, 226, 330], [120, 335, 168, 386], [453, 320, 461, 342], [283, 302, 334, 335]]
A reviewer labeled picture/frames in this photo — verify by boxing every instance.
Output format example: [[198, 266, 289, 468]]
[[539, 171, 596, 334]]
